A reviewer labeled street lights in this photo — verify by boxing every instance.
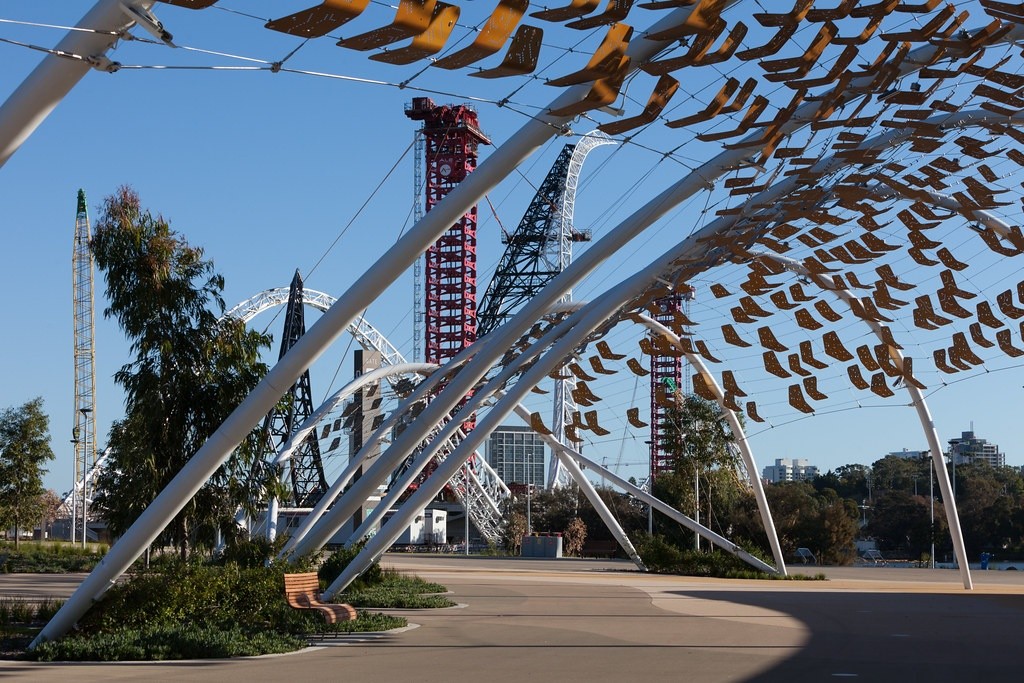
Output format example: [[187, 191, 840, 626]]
[[927, 455, 935, 569], [948, 441, 960, 570]]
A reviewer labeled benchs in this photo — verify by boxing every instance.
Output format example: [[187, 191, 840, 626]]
[[283, 572, 357, 641], [581, 540, 618, 560]]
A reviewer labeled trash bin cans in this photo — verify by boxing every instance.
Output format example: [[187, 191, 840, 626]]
[[520, 532, 562, 558], [980, 552, 989, 570]]
